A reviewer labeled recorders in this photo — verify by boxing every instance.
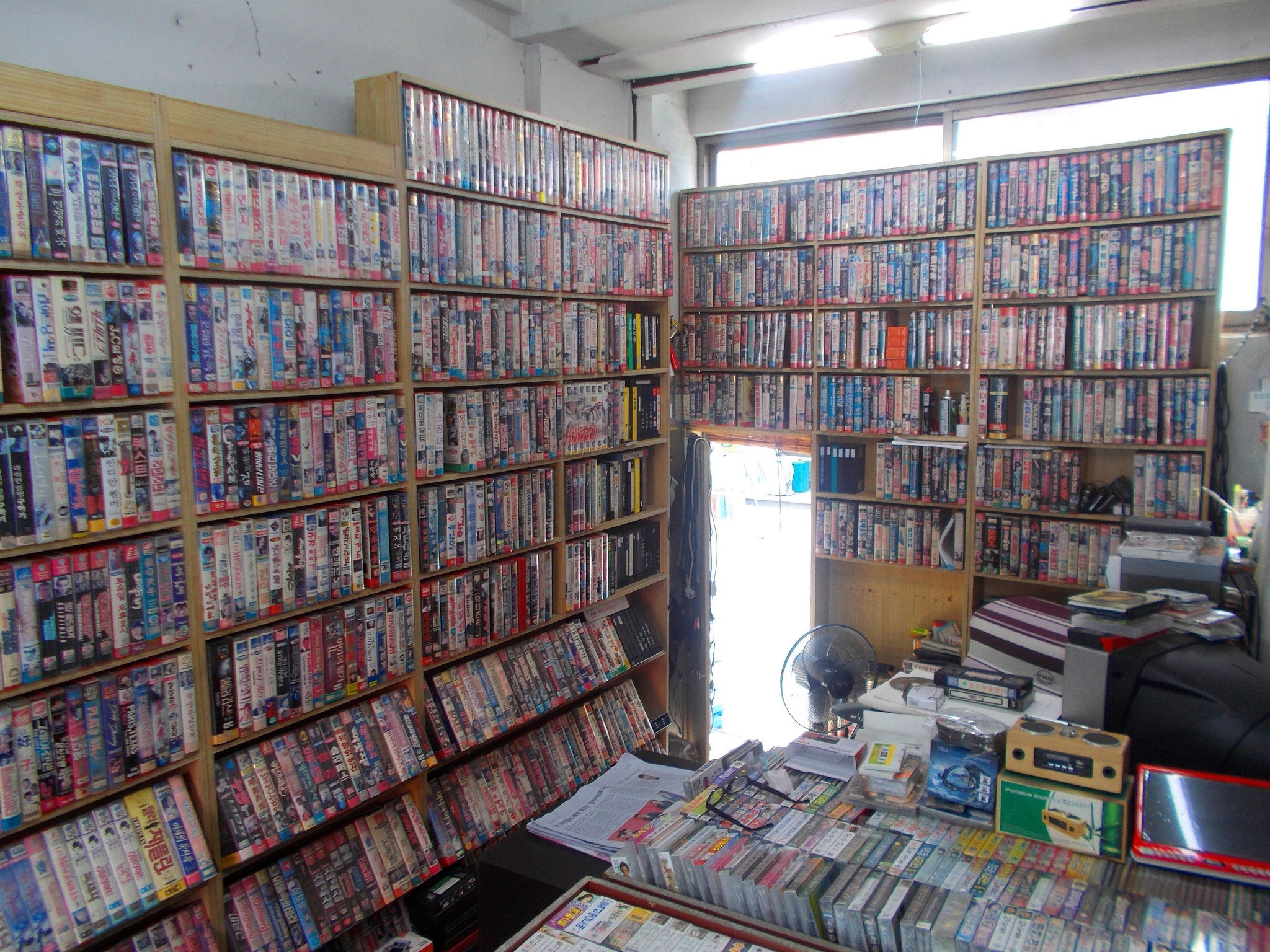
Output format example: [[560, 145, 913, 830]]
[[1004, 714, 1131, 795]]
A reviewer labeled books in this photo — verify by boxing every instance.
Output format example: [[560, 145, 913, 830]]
[[0, 88, 1270, 952]]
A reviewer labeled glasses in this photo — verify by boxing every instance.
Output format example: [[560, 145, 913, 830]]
[[705, 775, 810, 831]]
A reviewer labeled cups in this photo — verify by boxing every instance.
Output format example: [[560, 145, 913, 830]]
[[1225, 506, 1260, 564]]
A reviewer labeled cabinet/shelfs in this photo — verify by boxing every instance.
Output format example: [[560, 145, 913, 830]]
[[0, 61, 1232, 951]]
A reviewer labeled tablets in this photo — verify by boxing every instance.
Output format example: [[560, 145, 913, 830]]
[[1131, 765, 1269, 888]]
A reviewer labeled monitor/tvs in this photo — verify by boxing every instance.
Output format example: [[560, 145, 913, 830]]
[[1061, 632, 1269, 778]]
